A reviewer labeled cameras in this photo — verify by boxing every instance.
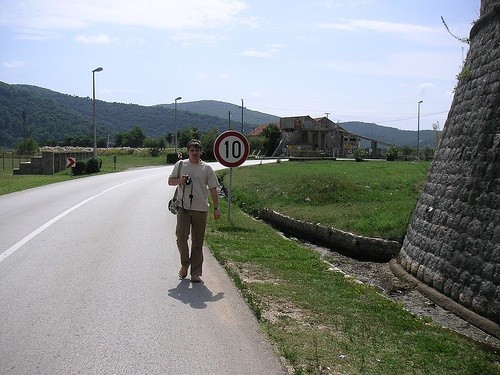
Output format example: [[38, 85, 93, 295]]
[[184, 176, 192, 185]]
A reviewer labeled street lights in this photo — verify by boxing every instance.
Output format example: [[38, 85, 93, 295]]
[[417, 100, 423, 162], [174, 96, 182, 154], [92, 66, 104, 171]]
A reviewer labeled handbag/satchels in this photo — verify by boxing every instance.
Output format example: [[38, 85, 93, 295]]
[[168, 198, 180, 214]]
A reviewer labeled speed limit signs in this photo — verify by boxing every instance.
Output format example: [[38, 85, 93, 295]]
[[213, 130, 250, 167]]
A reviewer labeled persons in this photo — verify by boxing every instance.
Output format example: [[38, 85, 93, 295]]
[[168, 139, 220, 281]]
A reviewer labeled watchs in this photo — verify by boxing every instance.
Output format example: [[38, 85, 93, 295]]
[[214, 207, 220, 209]]
